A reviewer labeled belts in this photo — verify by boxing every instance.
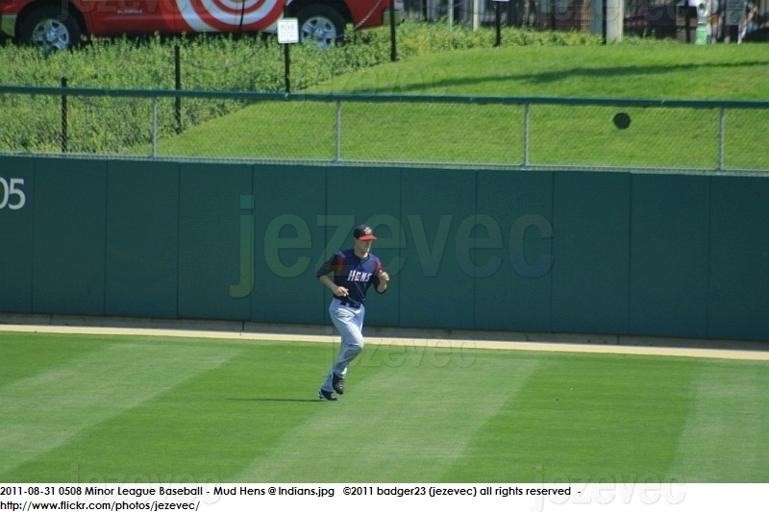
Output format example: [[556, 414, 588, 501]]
[[340, 301, 361, 308]]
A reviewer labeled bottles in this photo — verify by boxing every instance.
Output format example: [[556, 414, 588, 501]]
[[695, 21, 708, 46]]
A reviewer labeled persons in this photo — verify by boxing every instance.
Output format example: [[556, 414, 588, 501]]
[[316, 224, 390, 400]]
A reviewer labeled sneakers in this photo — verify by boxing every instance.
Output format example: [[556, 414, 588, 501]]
[[321, 370, 344, 401]]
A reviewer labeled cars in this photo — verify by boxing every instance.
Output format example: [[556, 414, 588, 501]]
[[1, 1, 390, 57]]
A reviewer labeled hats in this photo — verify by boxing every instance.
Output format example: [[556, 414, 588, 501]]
[[352, 224, 376, 241]]
